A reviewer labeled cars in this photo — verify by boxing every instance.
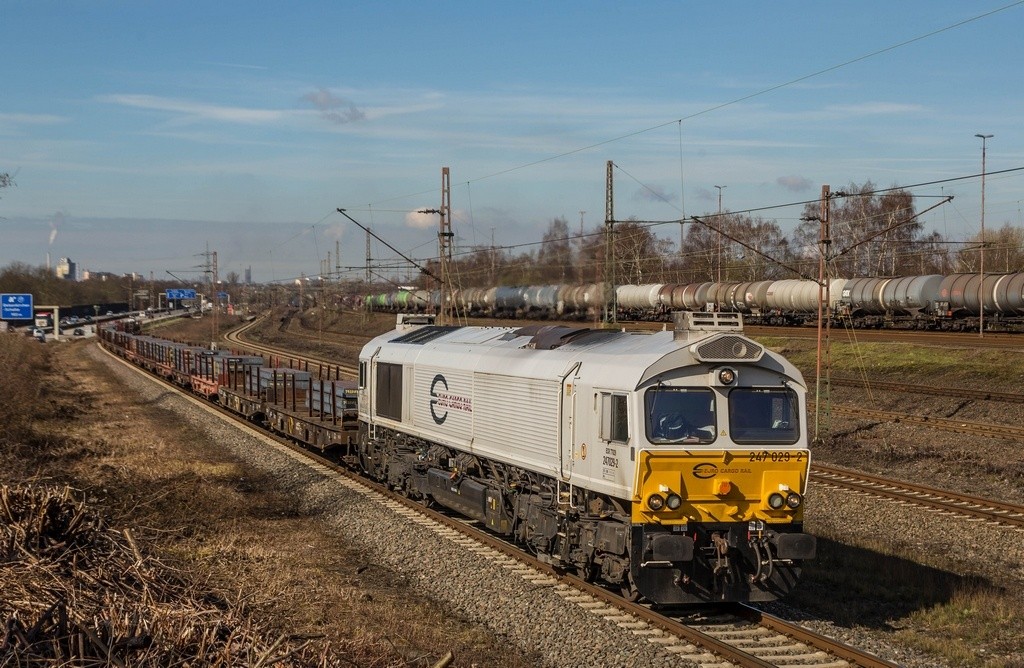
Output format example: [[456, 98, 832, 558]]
[[107, 305, 157, 321], [31, 312, 96, 342]]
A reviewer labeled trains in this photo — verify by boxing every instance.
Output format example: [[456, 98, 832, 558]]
[[356, 311, 818, 626]]
